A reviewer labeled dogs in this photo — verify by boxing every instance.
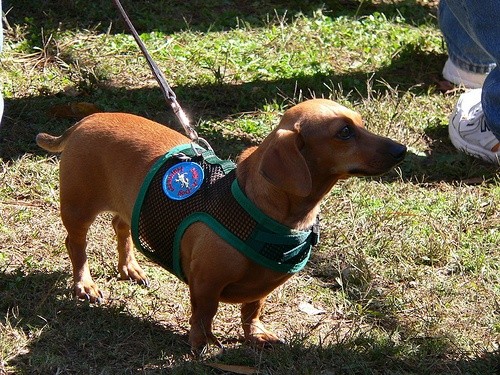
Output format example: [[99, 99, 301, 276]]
[[36, 97, 408, 359]]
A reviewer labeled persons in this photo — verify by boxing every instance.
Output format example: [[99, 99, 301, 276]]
[[438, 0, 500, 166]]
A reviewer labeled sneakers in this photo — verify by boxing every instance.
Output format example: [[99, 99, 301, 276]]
[[443, 57, 490, 88], [448, 89, 500, 165]]
